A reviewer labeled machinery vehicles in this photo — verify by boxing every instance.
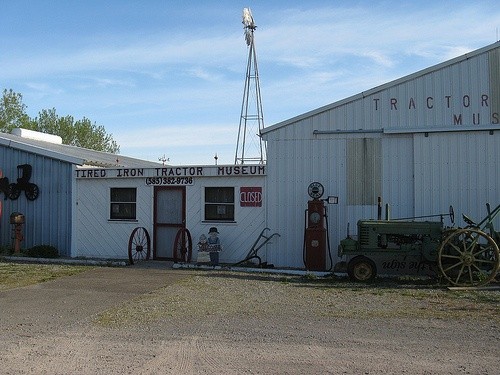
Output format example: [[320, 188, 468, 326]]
[[334, 196, 500, 288]]
